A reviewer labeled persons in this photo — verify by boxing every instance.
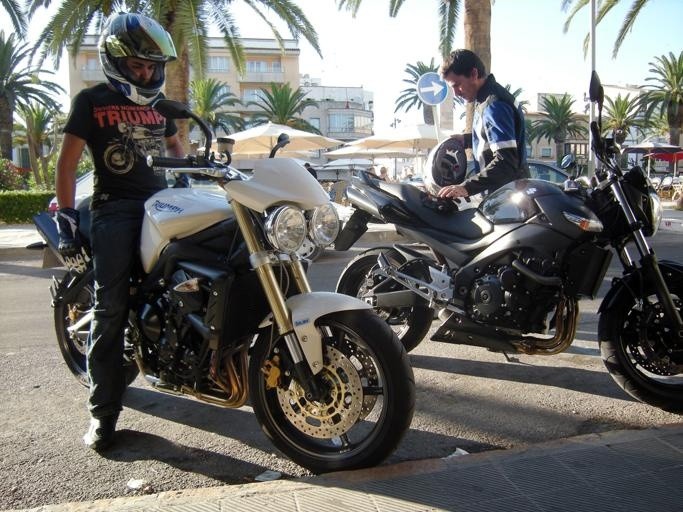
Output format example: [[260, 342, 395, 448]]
[[436, 48, 530, 200], [54, 12, 189, 448]]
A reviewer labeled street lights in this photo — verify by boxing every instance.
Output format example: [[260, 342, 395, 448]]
[[390, 117, 401, 182]]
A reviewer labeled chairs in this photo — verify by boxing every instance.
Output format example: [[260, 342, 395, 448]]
[[649, 175, 682, 203]]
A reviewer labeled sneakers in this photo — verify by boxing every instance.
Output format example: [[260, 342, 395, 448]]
[[83, 416, 116, 449]]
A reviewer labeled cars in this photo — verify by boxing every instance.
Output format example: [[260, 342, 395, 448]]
[[459, 158, 573, 204]]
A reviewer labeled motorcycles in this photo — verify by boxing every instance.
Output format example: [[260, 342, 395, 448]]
[[29, 94, 418, 479], [328, 68, 683, 417]]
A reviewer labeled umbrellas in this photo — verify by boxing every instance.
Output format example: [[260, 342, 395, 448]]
[[207, 121, 454, 183], [623, 141, 683, 178]]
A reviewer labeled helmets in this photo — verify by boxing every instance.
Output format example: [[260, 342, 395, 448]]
[[97, 13, 177, 106], [423, 138, 467, 198]]
